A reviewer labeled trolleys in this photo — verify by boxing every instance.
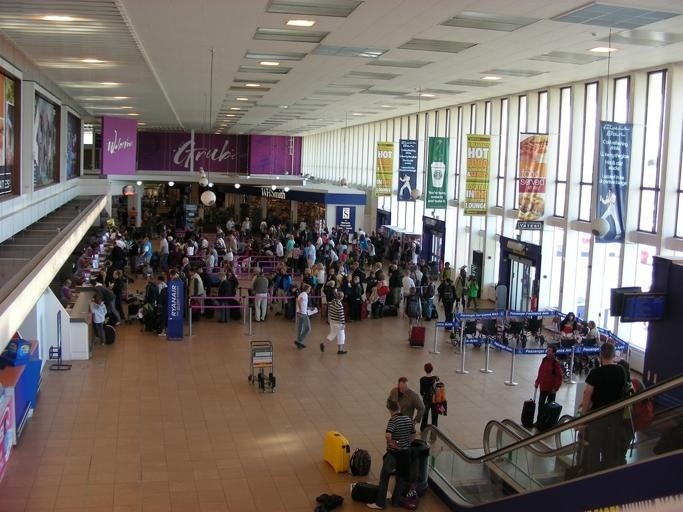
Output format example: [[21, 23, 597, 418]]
[[247, 338, 277, 393], [121, 271, 137, 303], [134, 254, 146, 278]]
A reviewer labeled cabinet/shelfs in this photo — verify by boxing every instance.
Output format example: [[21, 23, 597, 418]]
[[0, 331, 42, 446], [0, 387, 16, 482]]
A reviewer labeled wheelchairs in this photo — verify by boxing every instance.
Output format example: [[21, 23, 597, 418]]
[[558, 335, 580, 377], [577, 336, 601, 374], [449, 314, 548, 348]]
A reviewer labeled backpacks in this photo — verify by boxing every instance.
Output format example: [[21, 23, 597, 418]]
[[339, 274, 349, 291], [350, 448, 379, 503], [442, 286, 453, 303], [408, 299, 419, 318]]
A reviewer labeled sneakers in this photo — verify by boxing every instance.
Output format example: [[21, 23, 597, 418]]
[[367, 504, 383, 509]]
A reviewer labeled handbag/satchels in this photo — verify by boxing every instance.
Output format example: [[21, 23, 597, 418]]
[[378, 286, 390, 296], [402, 488, 419, 510], [628, 379, 654, 432], [432, 308, 438, 318], [429, 378, 447, 416]]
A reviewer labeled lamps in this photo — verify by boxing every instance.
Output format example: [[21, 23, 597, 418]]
[[199, 84, 217, 206]]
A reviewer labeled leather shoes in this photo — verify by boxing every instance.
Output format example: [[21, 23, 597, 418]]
[[320, 343, 325, 352], [337, 350, 347, 354]]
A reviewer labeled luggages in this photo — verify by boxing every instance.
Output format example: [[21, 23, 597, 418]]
[[410, 317, 426, 347], [565, 425, 588, 480], [104, 311, 117, 345], [521, 386, 562, 433], [322, 430, 351, 473], [228, 299, 241, 320]]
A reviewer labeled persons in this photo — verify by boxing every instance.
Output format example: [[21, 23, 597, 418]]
[[599, 188, 623, 240], [531, 346, 565, 428], [61, 207, 478, 336], [419, 363, 443, 432], [320, 292, 348, 353], [580, 320, 601, 346], [90, 295, 107, 344], [559, 312, 579, 349], [385, 377, 427, 441], [577, 343, 629, 476], [366, 402, 420, 510], [616, 358, 644, 468], [293, 285, 314, 348], [399, 173, 414, 201]]
